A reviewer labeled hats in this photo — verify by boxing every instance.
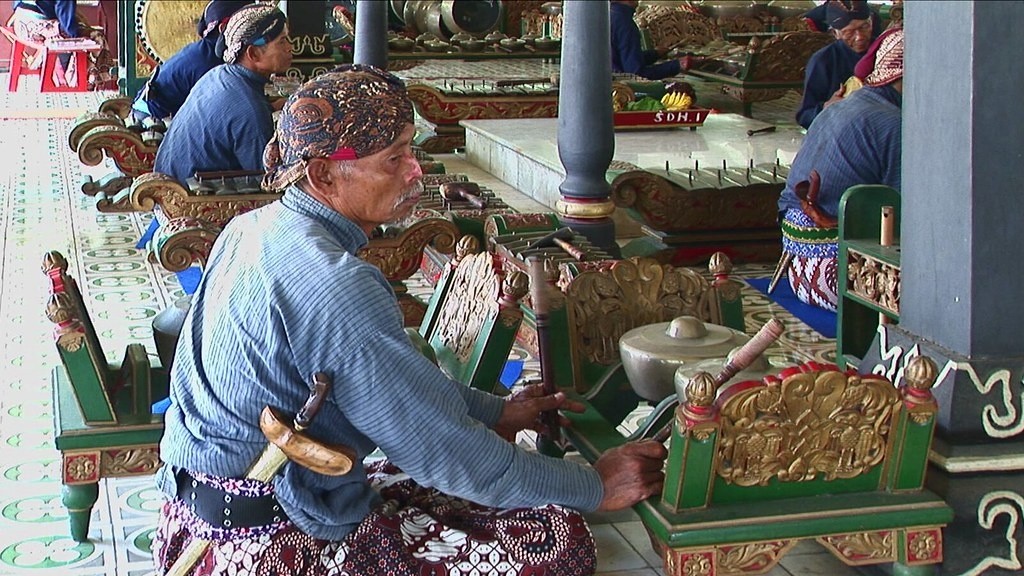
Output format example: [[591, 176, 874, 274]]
[[854, 26, 904, 87], [261, 64, 414, 193], [197, 0, 256, 39], [801, 0, 870, 33], [215, 2, 285, 65]]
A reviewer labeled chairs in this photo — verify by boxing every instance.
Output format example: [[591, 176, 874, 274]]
[[0, 26, 69, 92]]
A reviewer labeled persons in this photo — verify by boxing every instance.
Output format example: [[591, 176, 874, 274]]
[[774, 27, 904, 313], [129, 0, 244, 123], [151, 64, 668, 576], [13, 0, 81, 74], [795, 0, 883, 130], [153, 0, 294, 187], [608, 0, 705, 80]]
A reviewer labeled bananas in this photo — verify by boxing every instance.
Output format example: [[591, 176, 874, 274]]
[[660, 90, 692, 110]]
